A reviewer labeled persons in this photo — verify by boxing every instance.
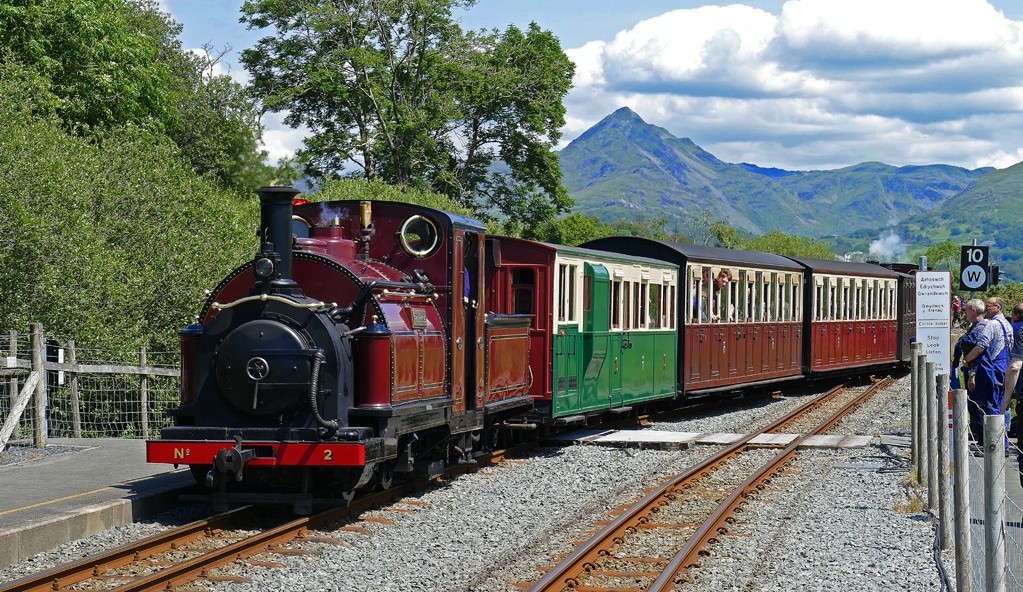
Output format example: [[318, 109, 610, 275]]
[[692, 269, 766, 323], [1000, 326, 1023, 525], [951, 295, 1023, 457], [712, 268, 733, 321]]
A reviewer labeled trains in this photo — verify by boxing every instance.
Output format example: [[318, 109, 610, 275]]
[[142, 179, 936, 516]]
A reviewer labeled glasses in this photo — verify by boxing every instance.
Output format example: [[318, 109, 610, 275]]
[[986, 305, 997, 307]]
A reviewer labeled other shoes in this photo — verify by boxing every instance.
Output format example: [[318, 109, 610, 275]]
[[970, 445, 984, 451], [973, 451, 984, 457]]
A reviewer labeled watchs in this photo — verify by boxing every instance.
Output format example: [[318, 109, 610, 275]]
[[963, 359, 968, 364]]
